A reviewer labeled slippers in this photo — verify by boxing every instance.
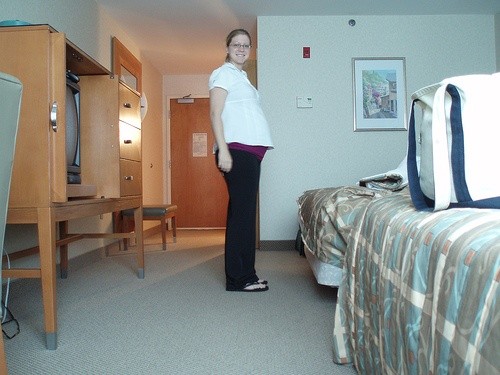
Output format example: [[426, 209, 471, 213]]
[[226, 282, 268, 291], [256, 280, 267, 284]]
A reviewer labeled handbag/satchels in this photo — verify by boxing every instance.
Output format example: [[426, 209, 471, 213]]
[[406, 71, 500, 212]]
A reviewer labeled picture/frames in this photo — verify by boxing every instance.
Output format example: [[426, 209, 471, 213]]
[[352, 57, 408, 132]]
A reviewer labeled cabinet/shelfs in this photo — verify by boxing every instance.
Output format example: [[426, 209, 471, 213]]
[[0, 26, 144, 349]]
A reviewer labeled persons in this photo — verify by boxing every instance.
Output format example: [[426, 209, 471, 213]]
[[208, 29, 275, 292]]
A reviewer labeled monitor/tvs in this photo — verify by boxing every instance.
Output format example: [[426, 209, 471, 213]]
[[66, 78, 81, 184]]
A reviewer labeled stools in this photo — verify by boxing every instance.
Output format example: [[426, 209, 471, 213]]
[[122, 204, 178, 250]]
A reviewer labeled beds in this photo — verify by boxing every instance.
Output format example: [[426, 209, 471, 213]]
[[298, 178, 500, 375]]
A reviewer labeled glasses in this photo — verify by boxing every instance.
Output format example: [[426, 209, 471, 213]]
[[232, 43, 251, 48]]
